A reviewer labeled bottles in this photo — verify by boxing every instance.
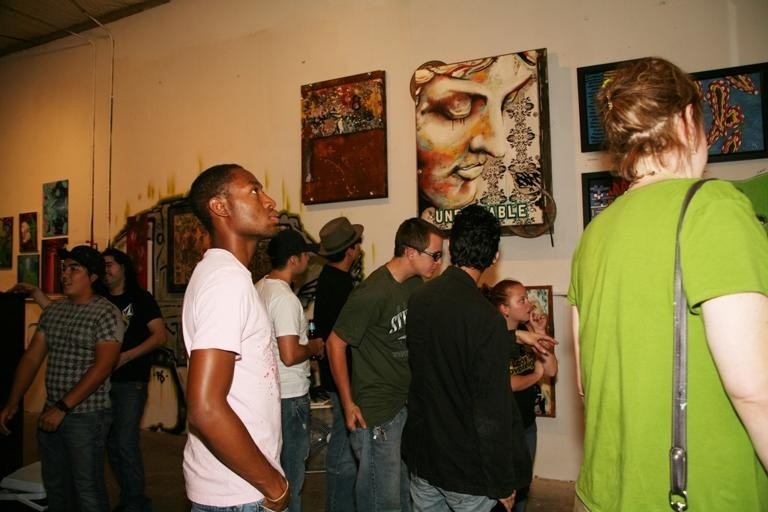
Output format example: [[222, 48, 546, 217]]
[[307, 318, 326, 397]]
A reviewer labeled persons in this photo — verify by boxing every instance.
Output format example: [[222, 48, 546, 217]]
[[180, 162, 291, 512], [412, 48, 542, 222], [564, 53, 768, 512], [476, 279, 557, 512], [310, 215, 366, 511], [5, 246, 168, 511], [255, 227, 328, 512], [18, 215, 38, 253], [322, 215, 449, 512], [1, 243, 129, 510], [400, 203, 559, 512]]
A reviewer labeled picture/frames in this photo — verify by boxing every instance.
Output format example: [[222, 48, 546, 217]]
[[166, 202, 207, 294], [683, 61, 768, 164], [580, 169, 624, 231], [575, 61, 619, 154], [516, 283, 558, 419]]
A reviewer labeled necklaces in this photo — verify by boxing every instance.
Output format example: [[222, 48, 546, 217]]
[[63, 293, 95, 329]]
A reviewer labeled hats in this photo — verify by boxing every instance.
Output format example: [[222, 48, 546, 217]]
[[318, 217, 363, 256], [59, 245, 104, 277], [266, 230, 318, 257]]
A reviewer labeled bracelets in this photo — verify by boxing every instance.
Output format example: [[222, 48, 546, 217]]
[[54, 397, 70, 415], [265, 477, 290, 502]]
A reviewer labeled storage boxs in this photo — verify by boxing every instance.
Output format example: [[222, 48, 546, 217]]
[[0, 459, 52, 512]]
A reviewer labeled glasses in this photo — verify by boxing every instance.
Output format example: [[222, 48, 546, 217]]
[[59, 264, 85, 275], [404, 244, 441, 262]]
